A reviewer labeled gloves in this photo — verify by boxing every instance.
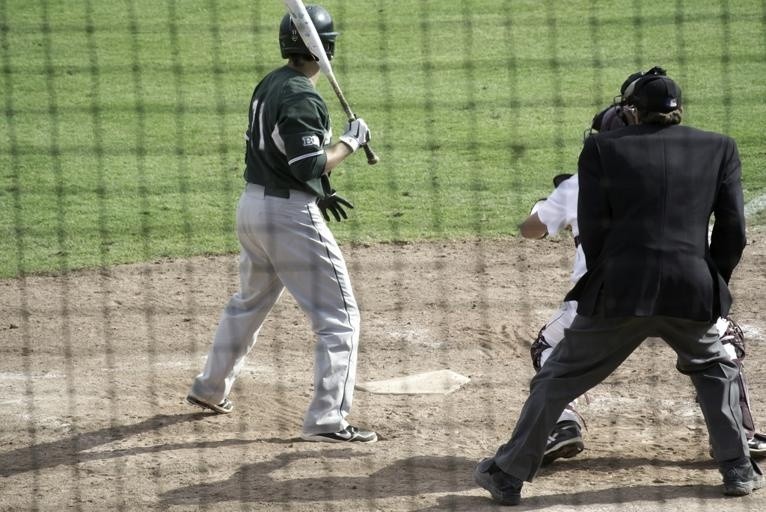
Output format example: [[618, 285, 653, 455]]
[[339, 117, 371, 153], [317, 188, 354, 222]]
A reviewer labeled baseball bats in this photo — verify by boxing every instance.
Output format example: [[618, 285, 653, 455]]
[[284, 0, 379, 164]]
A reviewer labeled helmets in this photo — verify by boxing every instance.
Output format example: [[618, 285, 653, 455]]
[[277, 4, 338, 61]]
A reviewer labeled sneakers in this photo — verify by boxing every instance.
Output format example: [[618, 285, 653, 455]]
[[744, 430, 766, 456], [539, 421, 584, 468], [474, 456, 523, 507], [300, 425, 378, 444], [186, 388, 233, 414], [721, 468, 766, 496]]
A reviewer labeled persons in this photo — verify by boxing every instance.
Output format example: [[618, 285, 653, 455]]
[[474, 67, 763, 506], [520, 172, 766, 466], [187, 7, 378, 446]]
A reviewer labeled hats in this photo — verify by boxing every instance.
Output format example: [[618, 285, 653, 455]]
[[583, 66, 684, 141]]
